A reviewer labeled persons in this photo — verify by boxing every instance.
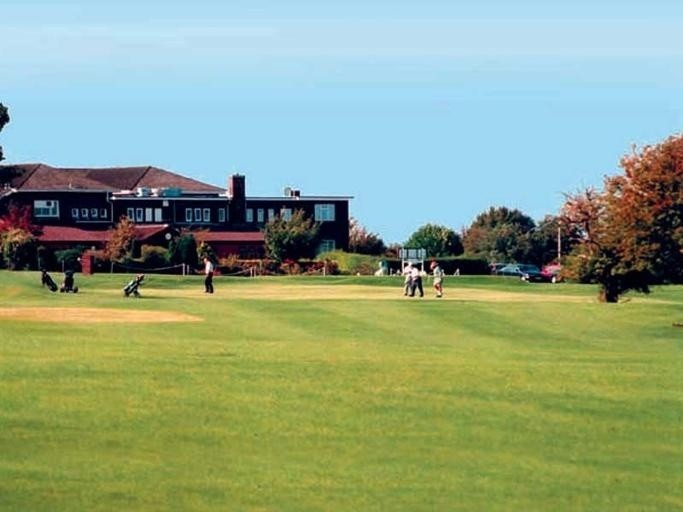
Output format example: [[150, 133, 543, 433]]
[[403, 264, 411, 295], [428, 261, 444, 298], [407, 262, 424, 298], [202, 257, 213, 293]]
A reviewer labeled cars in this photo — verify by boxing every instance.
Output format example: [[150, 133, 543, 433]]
[[489, 263, 563, 283]]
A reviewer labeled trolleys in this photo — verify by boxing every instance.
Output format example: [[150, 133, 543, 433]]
[[124, 274, 144, 298]]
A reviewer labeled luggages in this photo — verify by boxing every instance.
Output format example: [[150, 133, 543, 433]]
[[122, 272, 146, 298]]
